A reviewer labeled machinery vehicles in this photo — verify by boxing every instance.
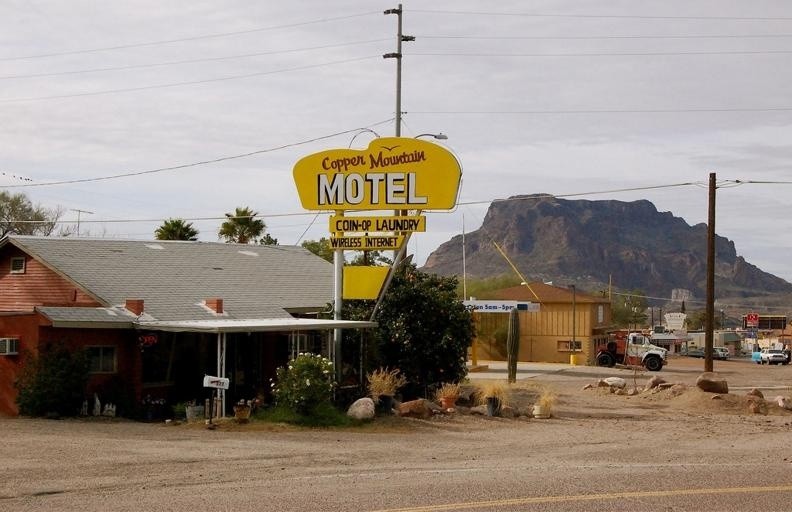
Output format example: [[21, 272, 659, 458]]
[[596, 332, 668, 371]]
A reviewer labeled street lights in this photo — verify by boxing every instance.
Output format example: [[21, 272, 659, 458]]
[[647, 306, 653, 331], [599, 289, 609, 298], [393, 134, 449, 284], [720, 309, 724, 330], [632, 306, 639, 330], [568, 284, 578, 366]]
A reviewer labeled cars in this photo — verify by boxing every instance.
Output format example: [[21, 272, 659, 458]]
[[684, 346, 730, 360], [759, 349, 785, 365]]
